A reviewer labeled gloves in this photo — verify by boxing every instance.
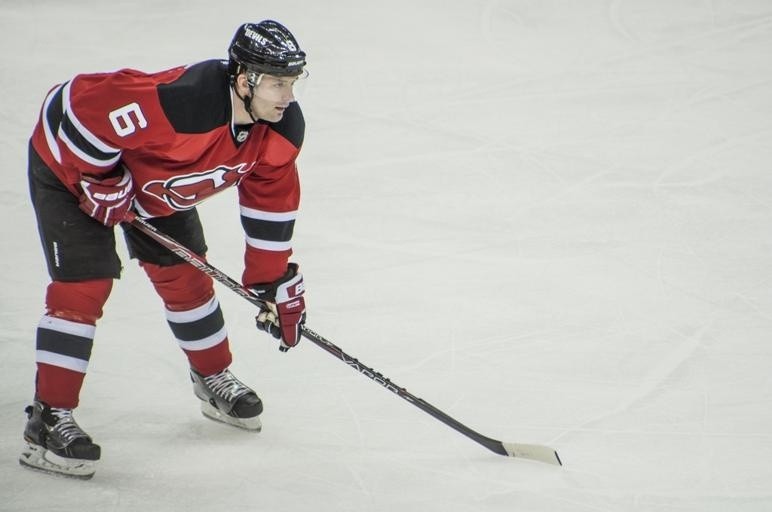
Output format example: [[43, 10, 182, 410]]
[[79, 166, 133, 227], [247, 263, 307, 353]]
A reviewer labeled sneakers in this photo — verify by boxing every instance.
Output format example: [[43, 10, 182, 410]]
[[190, 371, 262, 418], [24, 401, 100, 460]]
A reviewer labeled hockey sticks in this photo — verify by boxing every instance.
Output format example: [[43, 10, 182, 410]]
[[126, 212, 561, 464]]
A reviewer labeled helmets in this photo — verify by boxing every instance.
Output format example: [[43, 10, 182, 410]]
[[229, 20, 311, 101]]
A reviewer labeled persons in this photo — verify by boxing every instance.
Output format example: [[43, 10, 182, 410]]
[[23, 17, 310, 461]]
